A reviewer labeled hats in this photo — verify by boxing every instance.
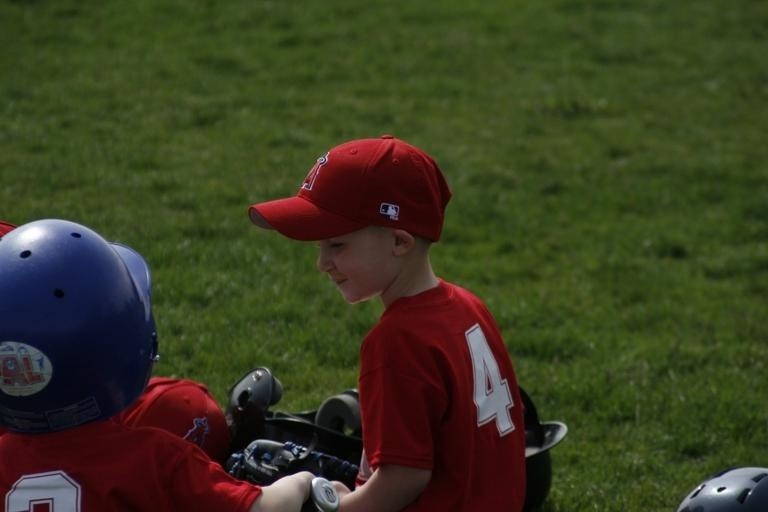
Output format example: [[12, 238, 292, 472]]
[[246, 132, 454, 244]]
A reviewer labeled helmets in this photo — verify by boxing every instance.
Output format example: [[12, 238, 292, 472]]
[[0, 215, 161, 438]]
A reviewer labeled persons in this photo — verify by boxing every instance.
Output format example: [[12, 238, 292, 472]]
[[247, 132, 528, 511], [0, 221, 237, 462], [0, 219, 316, 511], [668, 463, 768, 511]]
